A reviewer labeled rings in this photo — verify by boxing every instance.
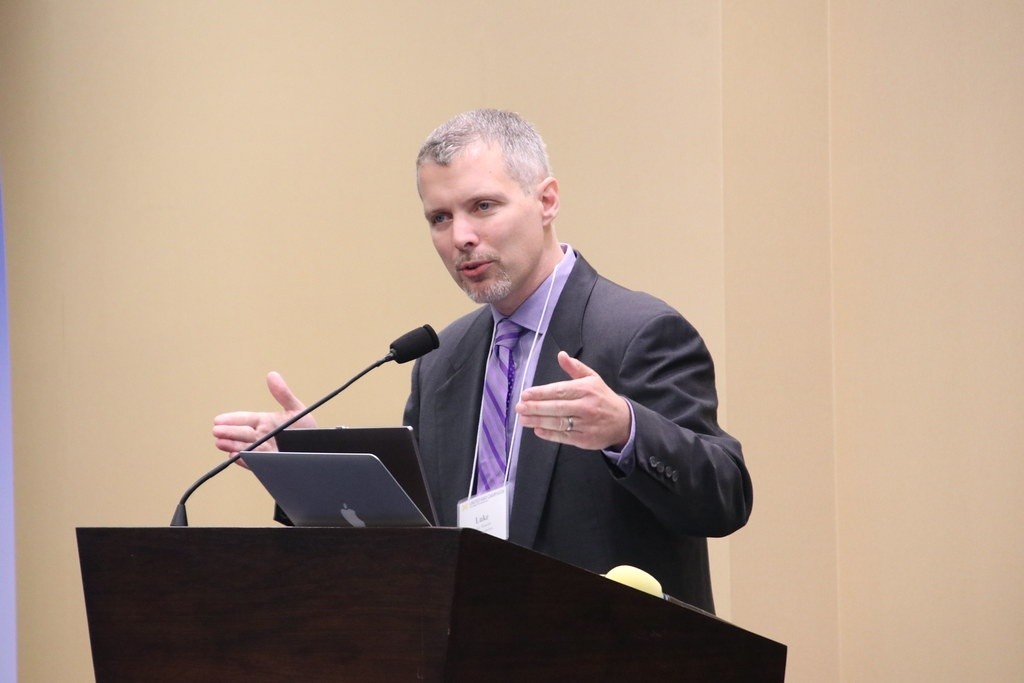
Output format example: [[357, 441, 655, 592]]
[[564, 418, 574, 433]]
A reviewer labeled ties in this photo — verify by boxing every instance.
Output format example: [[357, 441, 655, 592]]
[[475, 317, 522, 496]]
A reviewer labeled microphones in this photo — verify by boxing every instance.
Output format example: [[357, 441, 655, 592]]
[[606, 565, 725, 622], [169, 323, 440, 528]]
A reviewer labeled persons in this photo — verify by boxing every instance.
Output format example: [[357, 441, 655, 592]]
[[212, 110, 754, 613]]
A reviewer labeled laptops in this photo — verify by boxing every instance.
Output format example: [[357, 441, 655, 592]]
[[240, 426, 440, 529]]
